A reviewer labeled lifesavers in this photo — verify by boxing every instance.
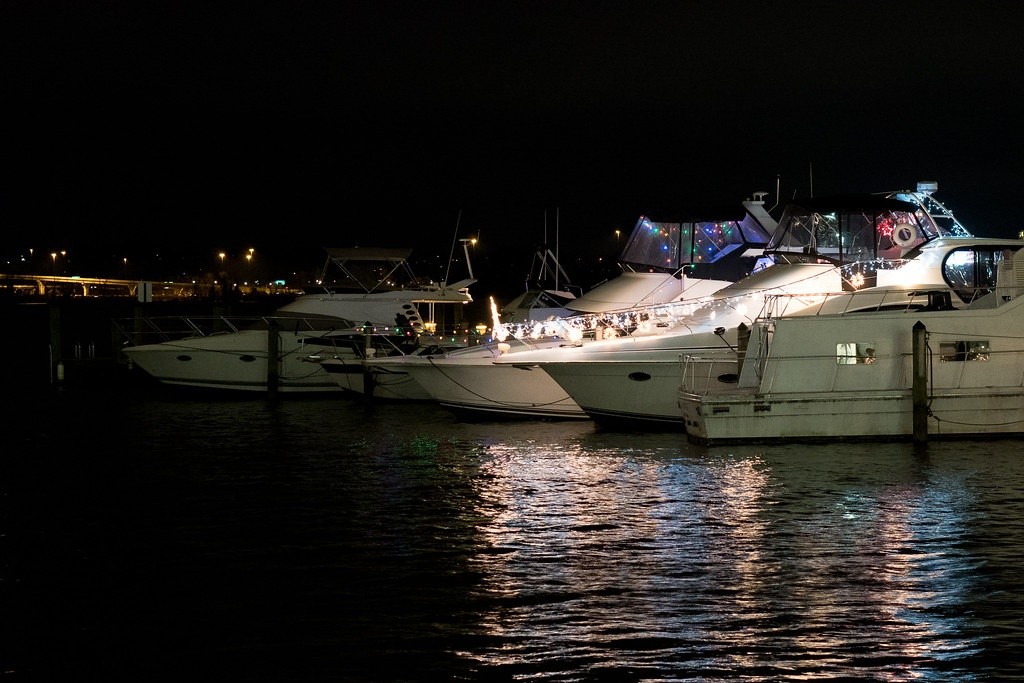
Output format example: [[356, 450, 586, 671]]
[[892, 223, 917, 247]]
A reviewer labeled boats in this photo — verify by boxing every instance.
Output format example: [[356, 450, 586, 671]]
[[0, 160, 1023, 444]]
